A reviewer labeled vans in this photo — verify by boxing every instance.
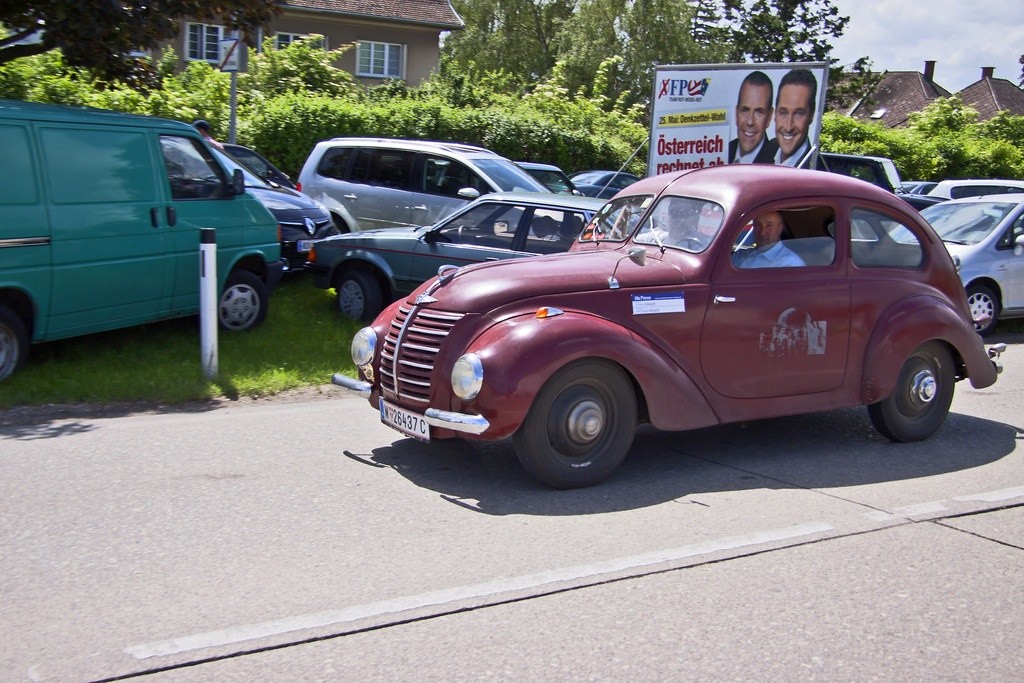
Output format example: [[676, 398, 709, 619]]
[[0, 103, 289, 387]]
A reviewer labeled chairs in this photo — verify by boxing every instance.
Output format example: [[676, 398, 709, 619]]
[[527, 216, 582, 254], [443, 164, 468, 194], [427, 161, 442, 194]]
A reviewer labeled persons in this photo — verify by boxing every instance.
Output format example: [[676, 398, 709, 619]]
[[760, 69, 817, 168], [728, 72, 774, 164], [733, 212, 806, 267], [193, 119, 224, 150], [609, 197, 711, 250]]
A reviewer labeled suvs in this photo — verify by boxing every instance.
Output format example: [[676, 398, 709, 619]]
[[298, 137, 588, 238], [801, 153, 956, 235]]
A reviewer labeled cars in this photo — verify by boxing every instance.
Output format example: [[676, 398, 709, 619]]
[[900, 181, 1024, 202], [887, 194, 1024, 332], [166, 146, 343, 268], [331, 165, 1006, 492], [545, 170, 641, 199], [426, 160, 585, 198], [304, 190, 653, 322]]
